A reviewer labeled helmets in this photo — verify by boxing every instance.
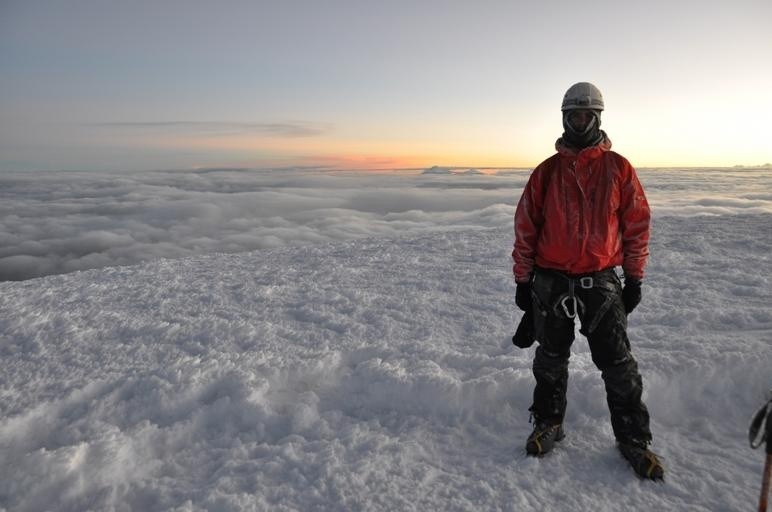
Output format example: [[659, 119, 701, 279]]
[[561, 82, 604, 111]]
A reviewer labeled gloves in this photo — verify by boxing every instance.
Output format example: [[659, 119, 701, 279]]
[[515, 283, 530, 312], [621, 279, 642, 317]]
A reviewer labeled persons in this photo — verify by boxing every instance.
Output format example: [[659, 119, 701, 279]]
[[510, 81, 664, 483]]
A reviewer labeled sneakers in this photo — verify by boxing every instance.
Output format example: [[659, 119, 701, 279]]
[[616, 439, 664, 481], [524, 420, 566, 455]]
